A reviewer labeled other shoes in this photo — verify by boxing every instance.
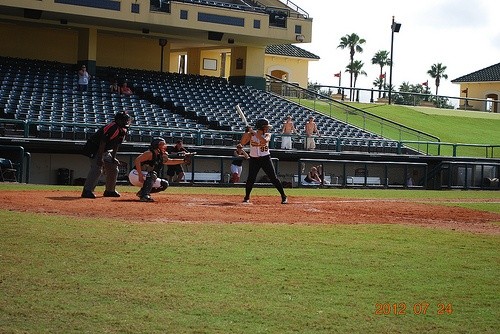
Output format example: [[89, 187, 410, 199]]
[[169, 182, 173, 186], [242, 197, 250, 203], [281, 196, 288, 204], [174, 183, 180, 186]]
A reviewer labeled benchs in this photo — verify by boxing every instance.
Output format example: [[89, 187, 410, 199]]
[[294, 175, 341, 186], [346, 176, 383, 187], [178, 172, 221, 183]]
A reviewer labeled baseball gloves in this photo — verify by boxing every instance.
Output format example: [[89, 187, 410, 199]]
[[185, 151, 196, 165]]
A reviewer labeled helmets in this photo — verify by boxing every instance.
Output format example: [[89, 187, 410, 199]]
[[150, 137, 167, 156], [236, 143, 244, 148], [255, 119, 273, 133], [115, 111, 134, 135]]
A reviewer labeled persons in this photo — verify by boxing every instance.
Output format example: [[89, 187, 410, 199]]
[[111, 80, 120, 94], [304, 166, 322, 183], [121, 81, 133, 95], [81, 112, 133, 198], [78, 64, 88, 91], [167, 139, 188, 183], [231, 144, 248, 182], [281, 115, 299, 149], [135, 137, 190, 202], [240, 118, 287, 204], [304, 116, 320, 151]]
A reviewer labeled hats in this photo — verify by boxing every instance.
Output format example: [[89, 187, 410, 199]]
[[286, 114, 292, 119], [177, 139, 181, 142]]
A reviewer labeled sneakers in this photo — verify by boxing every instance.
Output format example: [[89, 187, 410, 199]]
[[140, 195, 153, 202], [81, 190, 95, 198], [103, 190, 120, 197], [136, 192, 151, 201]]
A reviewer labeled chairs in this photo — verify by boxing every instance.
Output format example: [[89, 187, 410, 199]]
[[0, 159, 17, 182], [0, 0, 403, 148]]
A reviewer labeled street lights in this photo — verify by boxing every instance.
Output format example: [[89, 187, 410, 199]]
[[389, 16, 402, 104]]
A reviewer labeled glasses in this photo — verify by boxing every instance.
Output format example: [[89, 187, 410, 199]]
[[82, 67, 86, 68], [310, 117, 314, 120], [113, 82, 118, 84]]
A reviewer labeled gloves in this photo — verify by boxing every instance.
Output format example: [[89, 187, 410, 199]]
[[244, 152, 249, 158], [245, 126, 256, 136], [149, 171, 158, 182]]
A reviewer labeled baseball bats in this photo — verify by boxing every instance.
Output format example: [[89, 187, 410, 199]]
[[237, 106, 253, 136]]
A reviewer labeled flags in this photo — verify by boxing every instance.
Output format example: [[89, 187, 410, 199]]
[[462, 90, 467, 93], [379, 74, 385, 78], [423, 82, 428, 85], [334, 73, 340, 77]]
